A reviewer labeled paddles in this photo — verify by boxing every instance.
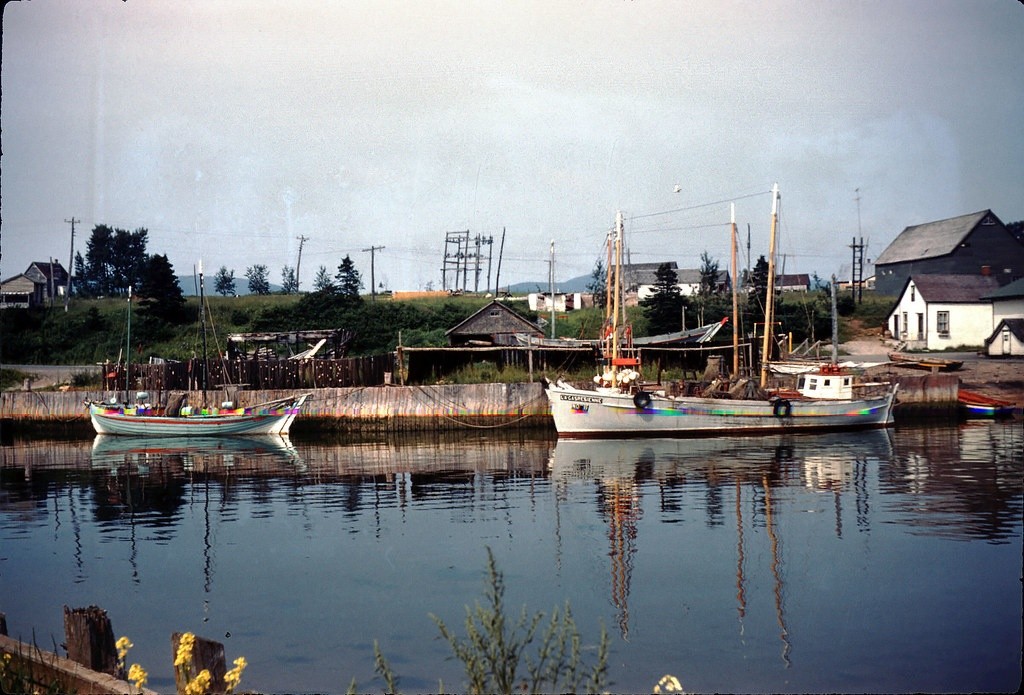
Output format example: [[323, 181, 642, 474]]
[[245, 392, 312, 410]]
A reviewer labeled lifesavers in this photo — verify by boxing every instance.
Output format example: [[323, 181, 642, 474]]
[[633, 391, 650, 409], [773, 399, 791, 419]]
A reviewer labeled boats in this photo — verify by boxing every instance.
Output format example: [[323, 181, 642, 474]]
[[887, 352, 965, 371], [957, 390, 1017, 419]]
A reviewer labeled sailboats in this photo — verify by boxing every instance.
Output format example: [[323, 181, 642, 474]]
[[90, 432, 306, 627], [550, 426, 895, 675], [544, 182, 899, 438], [83, 259, 314, 436]]
